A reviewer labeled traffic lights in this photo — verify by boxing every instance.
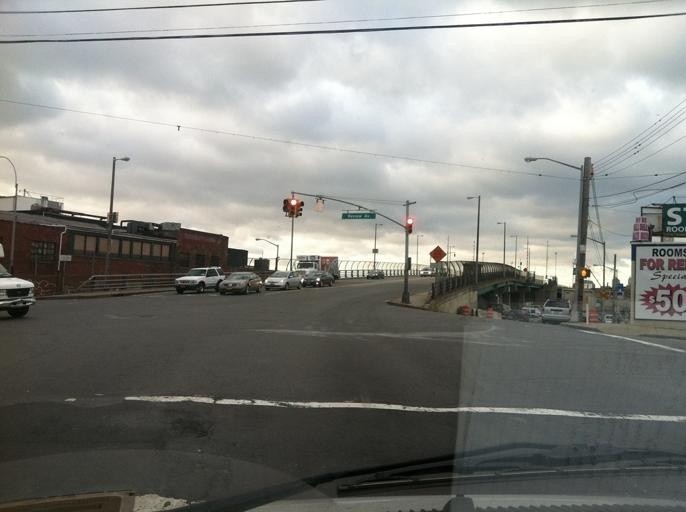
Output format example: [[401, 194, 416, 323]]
[[581, 268, 590, 278], [282, 198, 304, 217], [406, 217, 412, 234]]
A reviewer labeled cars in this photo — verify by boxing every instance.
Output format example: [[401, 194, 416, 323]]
[[419, 266, 437, 277], [366, 269, 384, 280], [264, 270, 302, 291], [520, 304, 542, 318], [540, 296, 572, 324], [300, 270, 335, 288], [486, 303, 512, 319], [0, 260, 36, 318], [218, 271, 263, 295]]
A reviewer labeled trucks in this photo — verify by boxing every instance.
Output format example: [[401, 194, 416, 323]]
[[296, 254, 339, 278]]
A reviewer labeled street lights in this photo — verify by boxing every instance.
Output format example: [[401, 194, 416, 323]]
[[497, 222, 506, 278], [570, 234, 606, 322], [102, 156, 130, 286], [256, 237, 279, 272], [466, 195, 481, 282], [524, 157, 591, 323]]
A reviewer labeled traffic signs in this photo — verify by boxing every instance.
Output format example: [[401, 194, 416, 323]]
[[341, 212, 376, 220]]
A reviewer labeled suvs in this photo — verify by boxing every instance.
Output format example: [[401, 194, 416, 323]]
[[174, 265, 225, 294]]
